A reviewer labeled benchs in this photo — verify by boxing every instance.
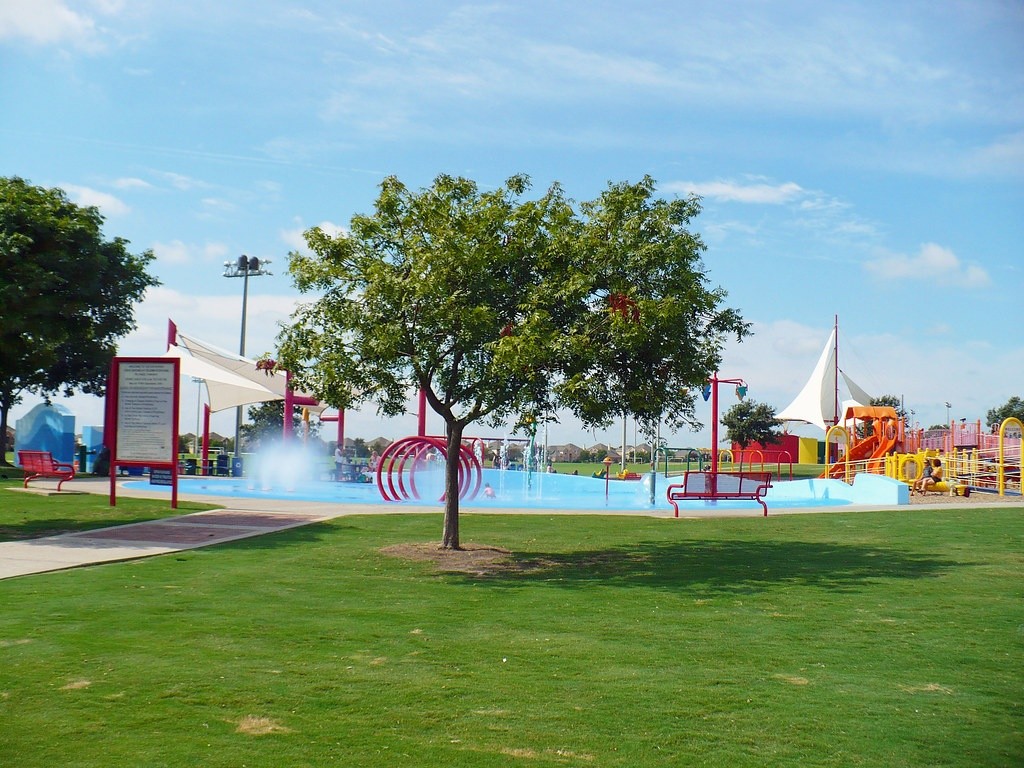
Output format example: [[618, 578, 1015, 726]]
[[18, 450, 75, 492], [194, 466, 237, 478], [667, 471, 774, 517]]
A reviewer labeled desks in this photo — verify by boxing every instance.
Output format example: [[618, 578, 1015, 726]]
[[203, 459, 220, 476]]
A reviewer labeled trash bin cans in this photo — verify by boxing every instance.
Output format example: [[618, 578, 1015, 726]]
[[231, 456, 244, 477], [216, 455, 229, 476], [182, 459, 197, 474]]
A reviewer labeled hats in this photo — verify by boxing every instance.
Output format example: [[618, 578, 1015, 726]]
[[923, 460, 929, 463]]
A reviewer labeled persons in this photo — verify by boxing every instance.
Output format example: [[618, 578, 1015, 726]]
[[368, 450, 381, 471], [335, 443, 346, 481], [910, 459, 933, 496], [918, 460, 942, 495], [546, 464, 554, 473], [479, 482, 496, 498]]
